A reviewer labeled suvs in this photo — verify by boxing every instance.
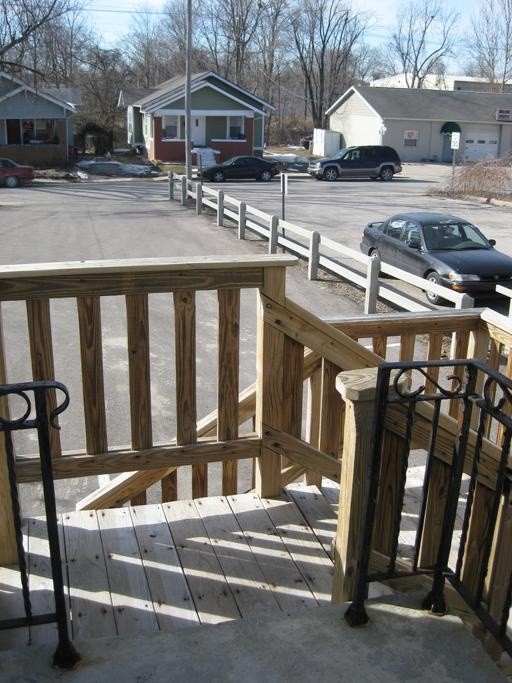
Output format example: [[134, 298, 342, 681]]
[[308, 143, 403, 182]]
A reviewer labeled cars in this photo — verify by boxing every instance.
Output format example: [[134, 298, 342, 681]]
[[1, 157, 35, 188], [74, 146, 160, 179], [299, 134, 313, 150], [197, 154, 280, 182], [359, 210, 511, 308]]
